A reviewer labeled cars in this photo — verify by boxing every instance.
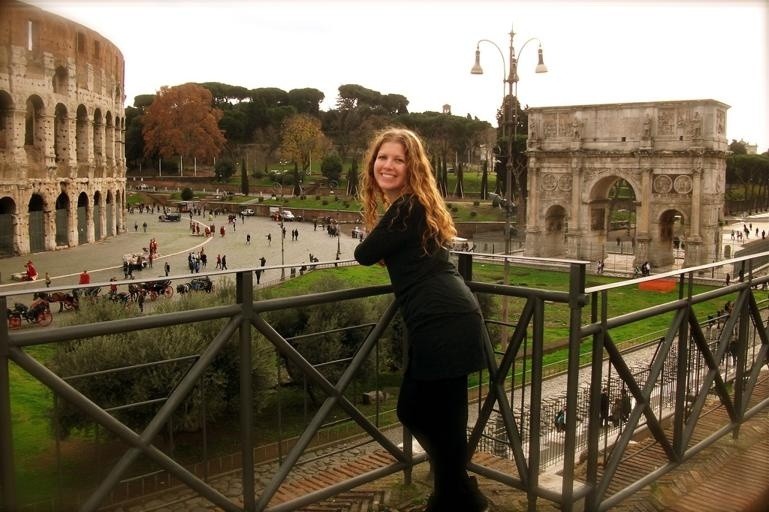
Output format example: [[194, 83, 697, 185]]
[[279, 211, 294, 222], [241, 209, 255, 215]]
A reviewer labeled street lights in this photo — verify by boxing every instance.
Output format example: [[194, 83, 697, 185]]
[[279, 160, 288, 199], [470, 21, 548, 456]]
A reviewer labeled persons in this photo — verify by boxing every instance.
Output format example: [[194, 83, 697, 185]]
[[596, 223, 766, 287], [45, 272, 52, 287], [359, 232, 363, 242], [729, 335, 739, 365], [556, 407, 584, 433], [465, 242, 468, 251], [610, 397, 621, 428], [352, 126, 498, 512], [618, 388, 633, 422], [79, 270, 89, 284], [468, 243, 476, 252], [460, 243, 464, 251], [723, 300, 733, 313], [598, 386, 611, 428], [109, 201, 341, 313]]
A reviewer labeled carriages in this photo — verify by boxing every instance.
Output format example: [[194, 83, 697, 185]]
[[127, 281, 173, 301], [6, 285, 101, 328]]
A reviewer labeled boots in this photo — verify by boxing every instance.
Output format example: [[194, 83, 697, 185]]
[[426, 469, 490, 512]]
